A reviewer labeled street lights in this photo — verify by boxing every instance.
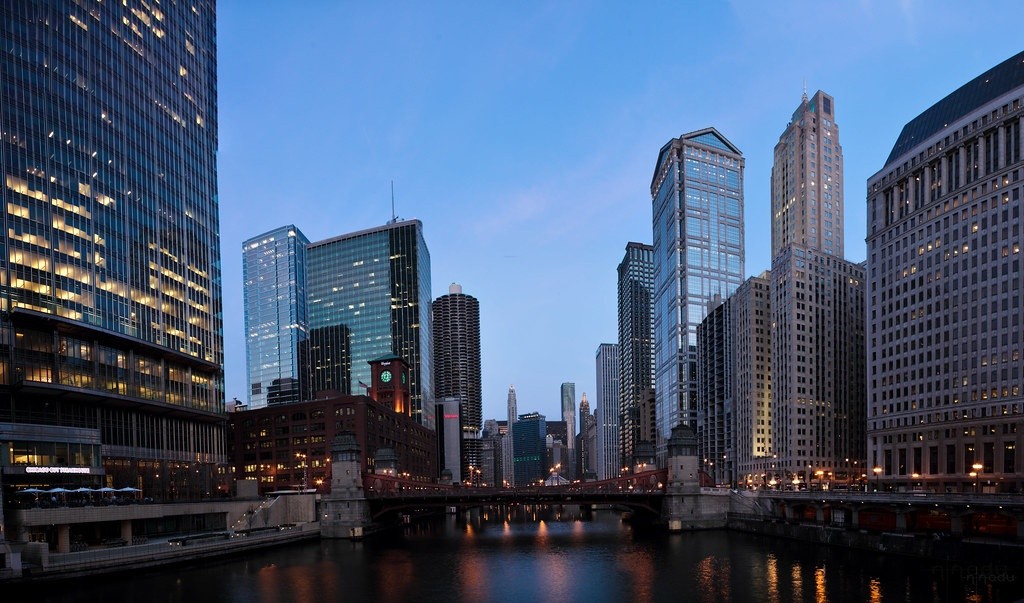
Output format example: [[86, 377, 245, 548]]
[[469, 466, 474, 484], [555, 464, 561, 486], [817, 471, 824, 490], [477, 470, 481, 488], [973, 462, 983, 499], [873, 468, 882, 493], [912, 474, 919, 490], [621, 467, 629, 477], [638, 463, 647, 492]]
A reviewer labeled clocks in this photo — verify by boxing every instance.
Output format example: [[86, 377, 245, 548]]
[[380, 370, 392, 382]]
